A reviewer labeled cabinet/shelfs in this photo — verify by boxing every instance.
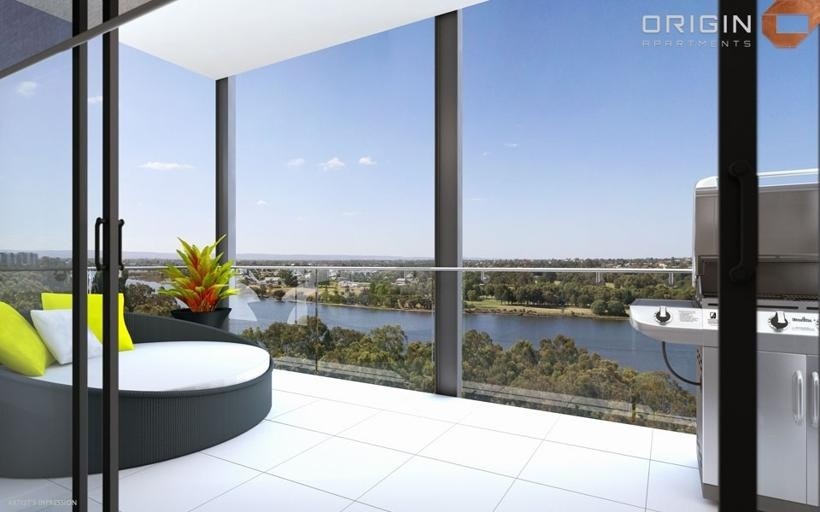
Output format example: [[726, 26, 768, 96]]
[[695, 346, 820, 512]]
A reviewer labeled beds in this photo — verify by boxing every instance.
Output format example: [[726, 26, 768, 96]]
[[0, 313, 275, 479]]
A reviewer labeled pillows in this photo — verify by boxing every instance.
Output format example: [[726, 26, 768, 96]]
[[0, 291, 134, 377]]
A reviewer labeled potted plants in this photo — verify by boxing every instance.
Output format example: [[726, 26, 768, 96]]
[[158, 233, 242, 328]]
